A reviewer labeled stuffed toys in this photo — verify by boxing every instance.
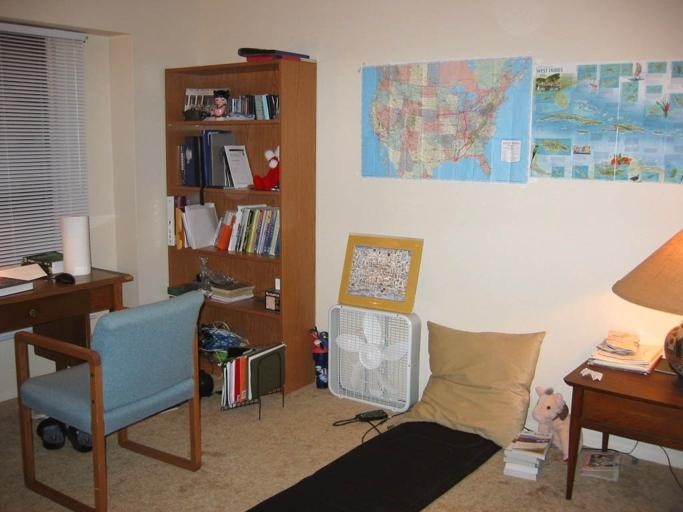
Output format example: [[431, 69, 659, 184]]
[[252, 145, 279, 189], [531, 385, 583, 463], [209, 89, 230, 120]]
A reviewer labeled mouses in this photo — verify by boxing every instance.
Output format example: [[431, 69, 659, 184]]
[[55, 271, 76, 286]]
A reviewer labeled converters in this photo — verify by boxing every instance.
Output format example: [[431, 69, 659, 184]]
[[358, 409, 387, 422]]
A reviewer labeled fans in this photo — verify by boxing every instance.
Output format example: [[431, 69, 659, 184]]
[[327, 304, 422, 412]]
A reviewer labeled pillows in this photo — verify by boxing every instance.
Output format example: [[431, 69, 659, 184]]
[[405, 320, 546, 451]]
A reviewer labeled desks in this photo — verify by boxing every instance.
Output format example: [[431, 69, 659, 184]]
[[563, 357, 683, 500], [0, 267, 134, 373]]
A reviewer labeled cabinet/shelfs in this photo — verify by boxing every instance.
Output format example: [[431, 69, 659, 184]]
[[165, 59, 317, 395]]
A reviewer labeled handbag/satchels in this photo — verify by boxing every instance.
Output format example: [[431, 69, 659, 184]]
[[198, 319, 249, 351]]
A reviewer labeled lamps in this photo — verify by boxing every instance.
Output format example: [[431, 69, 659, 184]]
[[611, 227, 683, 378]]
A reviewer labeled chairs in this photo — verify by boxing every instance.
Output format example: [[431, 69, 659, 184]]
[[14, 290, 205, 512]]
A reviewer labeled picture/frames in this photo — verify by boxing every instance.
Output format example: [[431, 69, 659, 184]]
[[338, 234, 424, 315]]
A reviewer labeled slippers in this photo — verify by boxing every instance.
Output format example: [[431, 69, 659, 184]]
[[36, 417, 66, 449], [68, 426, 92, 451]]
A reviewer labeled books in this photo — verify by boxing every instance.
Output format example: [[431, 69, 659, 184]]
[[503, 430, 553, 481], [167, 195, 219, 251], [211, 204, 281, 258], [580, 451, 620, 483], [219, 356, 248, 408], [231, 93, 279, 120], [208, 278, 256, 303], [587, 329, 664, 375], [177, 128, 253, 190], [0, 276, 35, 296]]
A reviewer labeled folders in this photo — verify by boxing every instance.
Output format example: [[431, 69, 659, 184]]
[[196, 136, 202, 187], [179, 145, 185, 185], [201, 129, 232, 187], [185, 136, 198, 186], [210, 134, 235, 187]]
